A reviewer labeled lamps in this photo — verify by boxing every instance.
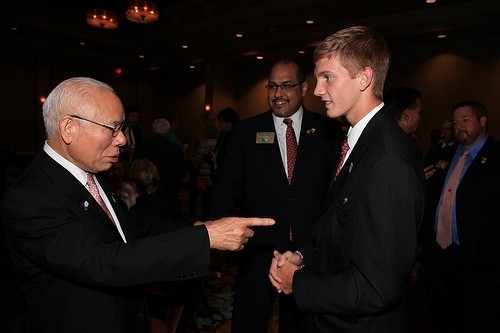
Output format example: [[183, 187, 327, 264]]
[[120, 0, 163, 26], [84, 6, 120, 32]]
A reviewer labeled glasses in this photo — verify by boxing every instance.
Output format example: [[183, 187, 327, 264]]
[[265, 83, 301, 90], [69, 115, 125, 137], [219, 120, 226, 123]]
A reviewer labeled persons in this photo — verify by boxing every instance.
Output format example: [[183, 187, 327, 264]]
[[0, 24, 500, 333]]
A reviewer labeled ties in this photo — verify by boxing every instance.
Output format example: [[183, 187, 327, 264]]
[[284, 118, 297, 240], [436, 151, 467, 249], [87, 175, 116, 226], [333, 136, 349, 181]]
